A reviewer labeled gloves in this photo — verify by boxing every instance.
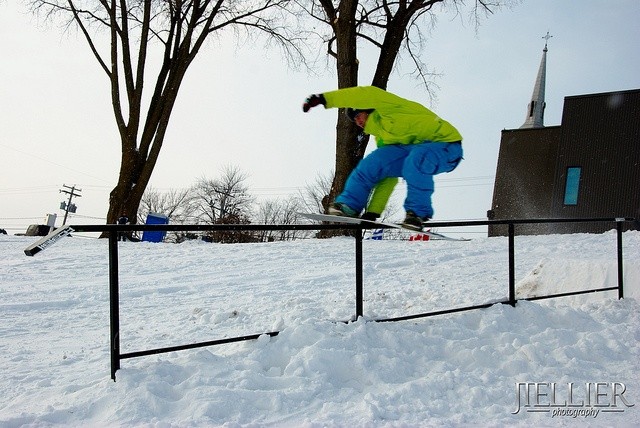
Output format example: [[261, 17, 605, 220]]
[[302, 93, 326, 113], [360, 211, 379, 221]]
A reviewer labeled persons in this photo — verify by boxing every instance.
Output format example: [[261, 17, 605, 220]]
[[302, 86, 463, 230]]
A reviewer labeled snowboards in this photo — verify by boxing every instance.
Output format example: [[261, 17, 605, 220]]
[[297, 211, 471, 242]]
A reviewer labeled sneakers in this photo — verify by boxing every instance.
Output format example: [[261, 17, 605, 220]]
[[327, 201, 345, 215], [403, 210, 423, 231]]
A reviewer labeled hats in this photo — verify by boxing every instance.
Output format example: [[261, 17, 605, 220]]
[[345, 106, 375, 123]]
